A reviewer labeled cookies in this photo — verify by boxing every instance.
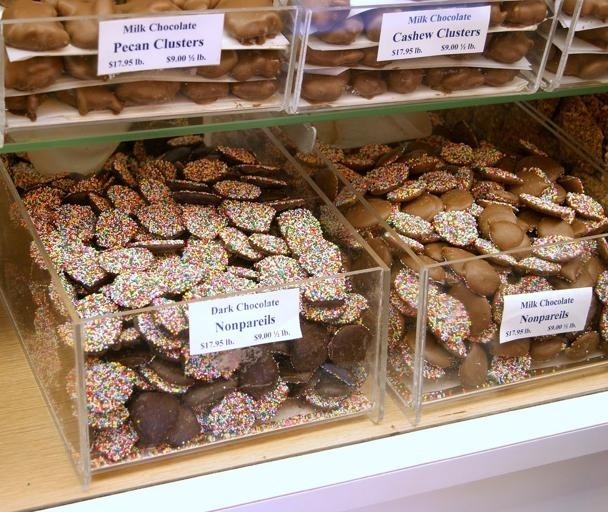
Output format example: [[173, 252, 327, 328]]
[[0, 92, 374, 469], [1, 2, 608, 121], [269, 100, 608, 401]]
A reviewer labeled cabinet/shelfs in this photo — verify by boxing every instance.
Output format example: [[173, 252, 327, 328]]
[[0, 0, 607, 512]]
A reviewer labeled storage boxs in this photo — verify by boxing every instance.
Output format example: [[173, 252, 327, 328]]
[[291, 2, 560, 108], [270, 101, 608, 422], [521, 0, 607, 94], [0, 2, 298, 127], [0, 125, 391, 482]]
